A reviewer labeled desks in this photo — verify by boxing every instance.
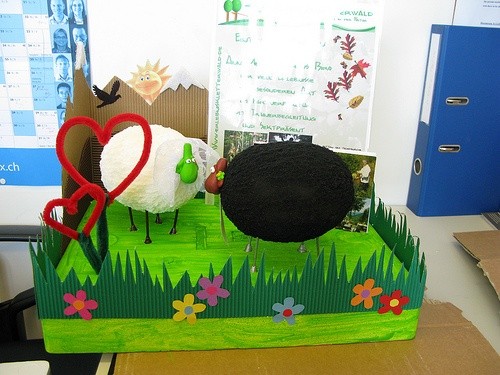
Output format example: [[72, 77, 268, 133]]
[[94, 204, 500, 374]]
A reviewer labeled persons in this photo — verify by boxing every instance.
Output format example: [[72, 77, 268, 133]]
[[55, 83, 74, 108], [352, 159, 371, 195], [72, 57, 89, 79], [47, 0, 68, 24], [72, 25, 88, 53], [54, 55, 72, 82], [68, 0, 87, 25], [58, 111, 66, 127], [50, 28, 70, 53]]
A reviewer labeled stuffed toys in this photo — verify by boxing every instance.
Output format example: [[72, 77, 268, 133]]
[[206, 142, 355, 274], [98, 125, 206, 244]]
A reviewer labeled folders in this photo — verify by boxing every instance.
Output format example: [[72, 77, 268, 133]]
[[407, 23, 500, 216]]
